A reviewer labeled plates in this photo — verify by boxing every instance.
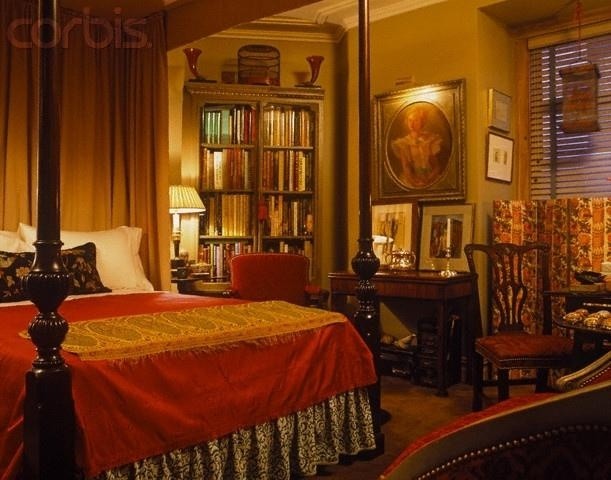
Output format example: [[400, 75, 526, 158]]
[[191, 271, 210, 278]]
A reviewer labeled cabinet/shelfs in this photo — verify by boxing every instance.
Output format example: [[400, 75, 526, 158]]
[[183, 82, 324, 285]]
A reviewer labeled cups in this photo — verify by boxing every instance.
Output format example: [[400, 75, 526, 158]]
[[177, 266, 189, 279]]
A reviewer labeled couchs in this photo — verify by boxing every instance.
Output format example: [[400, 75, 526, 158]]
[[378, 351, 611, 480]]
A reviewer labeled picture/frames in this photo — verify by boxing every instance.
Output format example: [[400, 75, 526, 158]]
[[372, 77, 467, 205], [416, 203, 476, 272], [372, 198, 417, 272], [485, 132, 514, 184]]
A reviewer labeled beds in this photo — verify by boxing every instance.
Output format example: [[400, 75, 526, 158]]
[[0, 221, 378, 480]]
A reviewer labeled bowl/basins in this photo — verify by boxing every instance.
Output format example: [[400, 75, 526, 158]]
[[191, 264, 213, 273]]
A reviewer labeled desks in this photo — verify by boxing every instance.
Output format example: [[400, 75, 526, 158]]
[[327, 269, 479, 398], [554, 308, 611, 369]]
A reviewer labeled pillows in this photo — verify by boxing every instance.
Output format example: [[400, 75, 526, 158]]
[[0, 241, 113, 307], [0, 230, 22, 252], [17, 222, 155, 294]]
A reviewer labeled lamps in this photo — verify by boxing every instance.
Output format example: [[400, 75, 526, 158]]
[[169, 184, 207, 269]]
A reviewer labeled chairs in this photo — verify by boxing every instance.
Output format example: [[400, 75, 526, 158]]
[[463, 242, 575, 404], [222, 253, 330, 313]]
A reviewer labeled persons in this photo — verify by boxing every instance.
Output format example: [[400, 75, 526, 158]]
[[391, 104, 444, 187], [437, 222, 455, 256]]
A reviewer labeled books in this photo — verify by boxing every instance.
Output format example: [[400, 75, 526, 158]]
[[203, 105, 256, 146], [263, 105, 314, 147], [198, 239, 253, 284], [261, 194, 314, 237], [261, 150, 313, 194], [262, 240, 315, 281], [201, 194, 251, 237], [201, 148, 253, 190]]
